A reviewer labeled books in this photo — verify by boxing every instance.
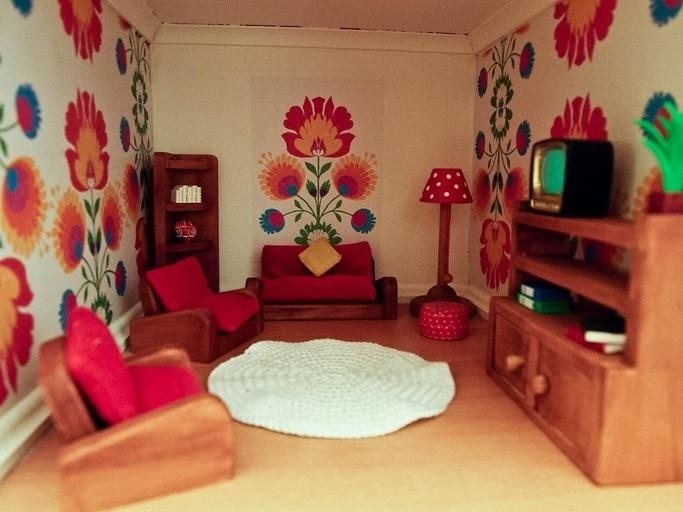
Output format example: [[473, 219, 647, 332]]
[[585, 313, 627, 344], [565, 324, 623, 354], [517, 293, 567, 314], [521, 284, 570, 300]]
